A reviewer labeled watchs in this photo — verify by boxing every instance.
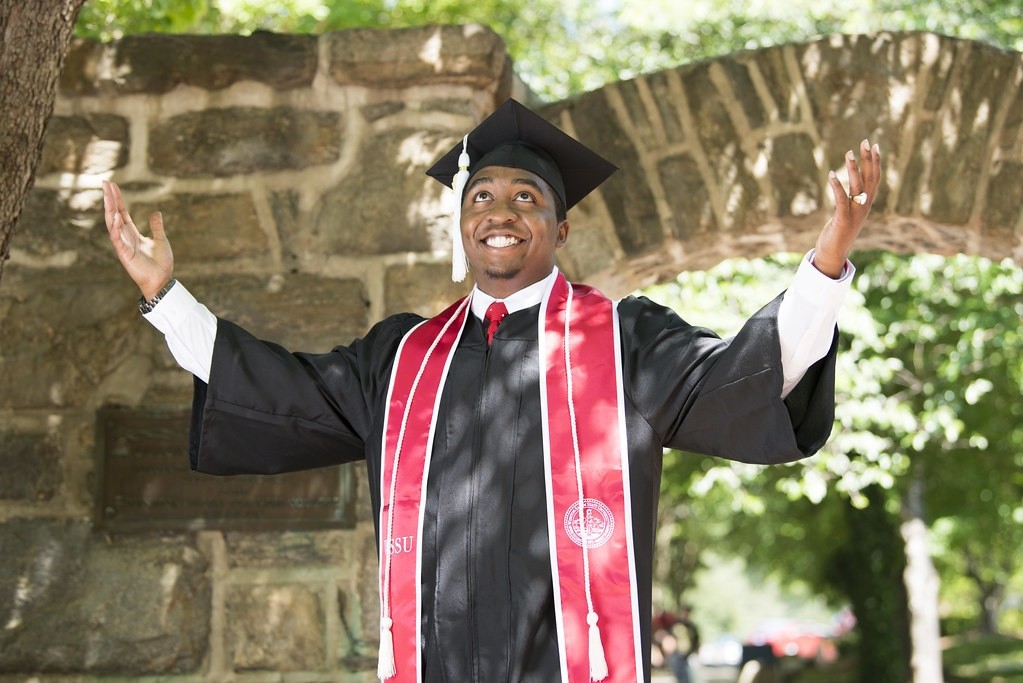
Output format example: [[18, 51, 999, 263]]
[[134, 279, 176, 314]]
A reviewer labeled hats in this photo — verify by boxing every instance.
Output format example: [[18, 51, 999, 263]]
[[425, 97, 619, 282]]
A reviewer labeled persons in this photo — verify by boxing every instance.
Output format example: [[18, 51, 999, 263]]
[[99, 96, 882, 683]]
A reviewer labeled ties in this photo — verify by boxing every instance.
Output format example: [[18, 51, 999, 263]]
[[485, 301, 509, 347]]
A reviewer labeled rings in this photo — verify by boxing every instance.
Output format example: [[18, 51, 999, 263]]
[[848, 189, 867, 208]]
